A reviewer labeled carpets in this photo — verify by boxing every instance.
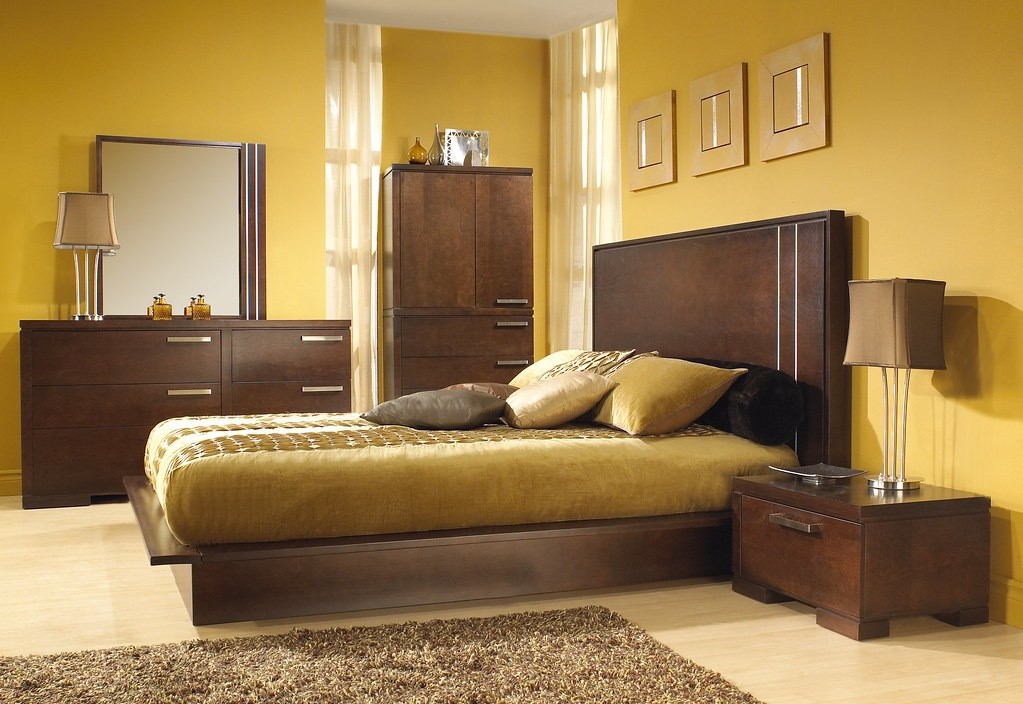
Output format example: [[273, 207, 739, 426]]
[[0, 603, 765, 704]]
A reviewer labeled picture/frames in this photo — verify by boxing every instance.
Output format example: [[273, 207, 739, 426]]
[[692, 61, 748, 176], [631, 88, 677, 192], [759, 32, 829, 162]]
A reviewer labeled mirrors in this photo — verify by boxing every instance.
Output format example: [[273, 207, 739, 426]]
[[95, 135, 267, 320]]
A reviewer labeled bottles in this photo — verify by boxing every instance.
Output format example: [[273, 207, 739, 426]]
[[408, 136, 429, 165], [427, 120, 444, 165]]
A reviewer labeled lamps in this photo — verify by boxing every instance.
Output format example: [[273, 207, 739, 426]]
[[53, 191, 120, 321], [842, 276, 948, 489]]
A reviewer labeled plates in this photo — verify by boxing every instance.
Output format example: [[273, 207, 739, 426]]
[[769, 464, 867, 479]]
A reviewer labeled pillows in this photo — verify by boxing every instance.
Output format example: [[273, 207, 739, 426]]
[[439, 382, 517, 425], [509, 349, 637, 389], [587, 356, 747, 437], [361, 388, 505, 430], [500, 371, 620, 429]]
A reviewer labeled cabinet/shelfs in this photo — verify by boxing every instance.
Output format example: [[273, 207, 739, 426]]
[[382, 164, 534, 402], [19, 320, 351, 510]]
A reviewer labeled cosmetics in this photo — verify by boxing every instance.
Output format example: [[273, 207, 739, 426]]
[[148, 296, 160, 315], [183, 297, 197, 316], [192, 294, 211, 320], [152, 293, 173, 321]]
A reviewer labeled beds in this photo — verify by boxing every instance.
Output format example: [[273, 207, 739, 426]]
[[121, 210, 850, 628]]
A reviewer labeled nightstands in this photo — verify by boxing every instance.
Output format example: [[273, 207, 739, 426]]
[[730, 472, 991, 641]]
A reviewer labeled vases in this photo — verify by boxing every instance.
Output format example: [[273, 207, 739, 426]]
[[428, 123, 447, 164]]
